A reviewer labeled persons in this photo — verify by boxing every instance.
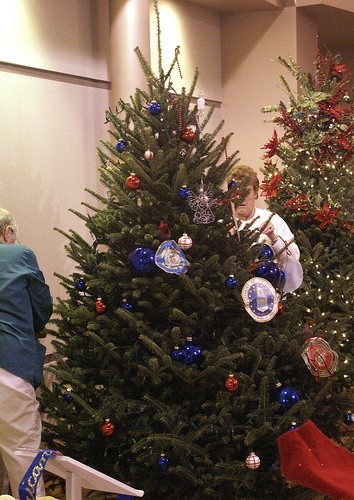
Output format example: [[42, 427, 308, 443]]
[[221, 163, 306, 295], [1, 207, 54, 499]]
